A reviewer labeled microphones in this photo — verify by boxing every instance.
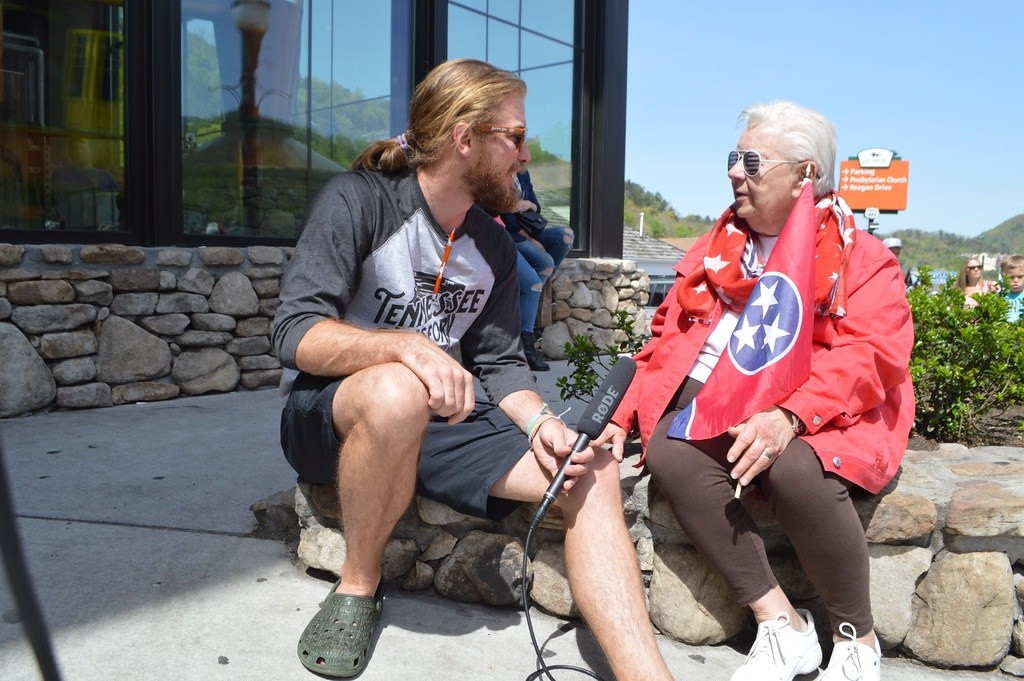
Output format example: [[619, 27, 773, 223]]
[[527, 357, 637, 530]]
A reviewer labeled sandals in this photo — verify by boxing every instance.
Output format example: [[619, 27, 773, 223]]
[[297, 578, 385, 678]]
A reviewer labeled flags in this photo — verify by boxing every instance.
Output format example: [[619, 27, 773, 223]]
[[667, 178, 817, 441]]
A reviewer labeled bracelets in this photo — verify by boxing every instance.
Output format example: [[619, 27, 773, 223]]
[[527, 402, 572, 447]]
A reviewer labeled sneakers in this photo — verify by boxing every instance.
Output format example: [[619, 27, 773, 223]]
[[812, 622, 882, 681], [730, 608, 823, 681]]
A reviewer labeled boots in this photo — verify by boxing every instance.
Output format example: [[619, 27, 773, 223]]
[[520, 331, 550, 371]]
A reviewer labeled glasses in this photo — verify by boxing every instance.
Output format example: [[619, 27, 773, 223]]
[[471, 123, 528, 153], [727, 149, 800, 178], [966, 265, 984, 272]]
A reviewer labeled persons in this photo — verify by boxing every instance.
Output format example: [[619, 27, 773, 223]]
[[51, 138, 125, 231], [998, 256, 1023, 323], [494, 163, 575, 372], [585, 100, 916, 681], [882, 237, 901, 258], [952, 259, 1002, 312], [270, 58, 676, 681]]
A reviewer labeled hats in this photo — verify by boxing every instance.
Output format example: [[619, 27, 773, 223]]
[[882, 237, 902, 248]]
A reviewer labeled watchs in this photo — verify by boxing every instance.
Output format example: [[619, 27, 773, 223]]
[[792, 413, 805, 435]]
